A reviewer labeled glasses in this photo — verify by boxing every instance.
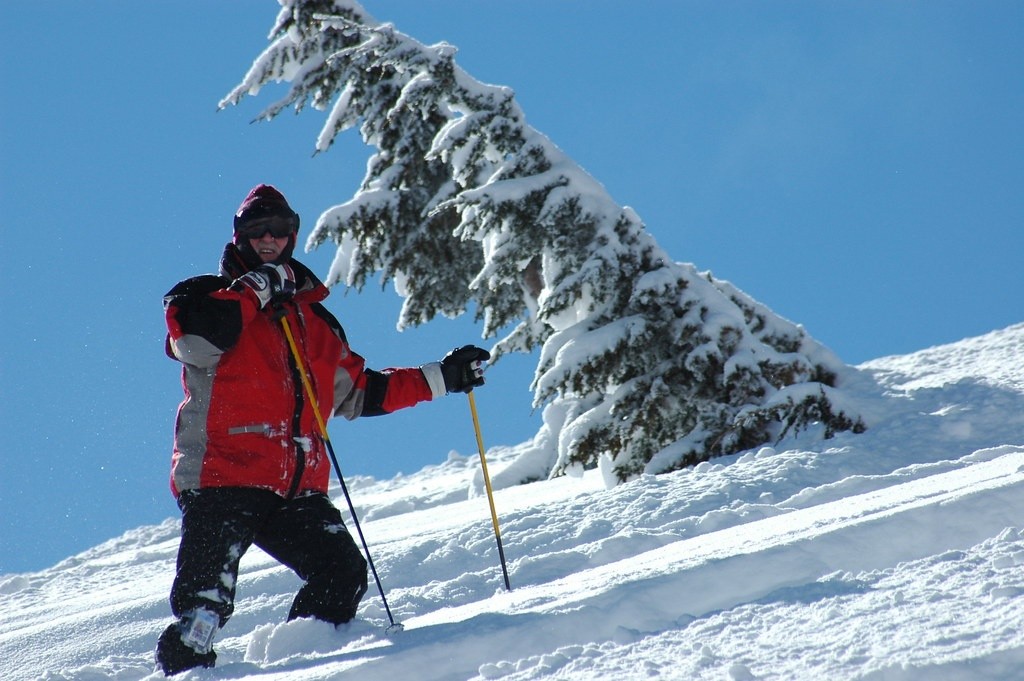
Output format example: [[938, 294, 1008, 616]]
[[245, 222, 294, 239]]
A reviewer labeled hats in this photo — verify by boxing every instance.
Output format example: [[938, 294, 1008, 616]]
[[232, 183, 297, 247]]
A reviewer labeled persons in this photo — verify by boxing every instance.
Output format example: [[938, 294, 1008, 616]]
[[194, 622, 203, 633], [154, 184, 491, 677]]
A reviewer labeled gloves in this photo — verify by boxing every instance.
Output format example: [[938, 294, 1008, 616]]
[[230, 259, 297, 311], [438, 344, 491, 394]]
